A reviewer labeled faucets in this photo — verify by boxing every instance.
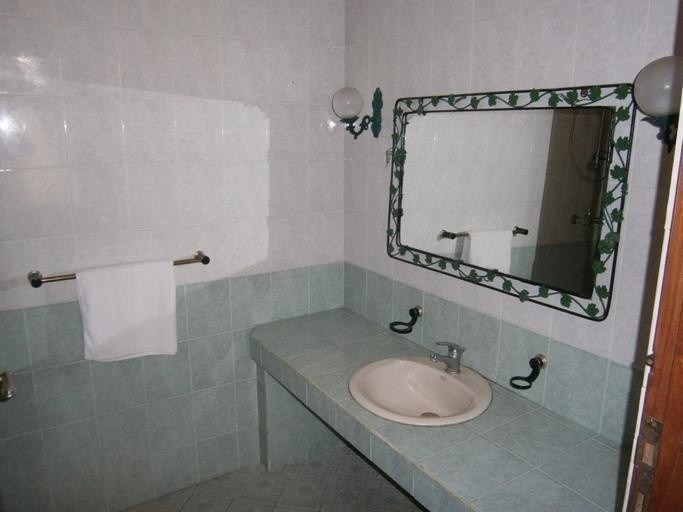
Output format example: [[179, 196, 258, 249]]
[[430, 341, 465, 374]]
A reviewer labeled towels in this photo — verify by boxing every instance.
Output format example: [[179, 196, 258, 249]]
[[75, 259, 178, 363], [462, 231, 512, 276]]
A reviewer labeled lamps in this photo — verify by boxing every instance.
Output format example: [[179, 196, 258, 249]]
[[332, 87, 383, 141], [632, 55, 683, 156]]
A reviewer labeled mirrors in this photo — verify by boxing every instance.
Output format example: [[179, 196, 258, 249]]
[[384, 83, 637, 321]]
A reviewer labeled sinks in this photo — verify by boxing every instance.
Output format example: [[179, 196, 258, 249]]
[[349, 356, 494, 427]]
